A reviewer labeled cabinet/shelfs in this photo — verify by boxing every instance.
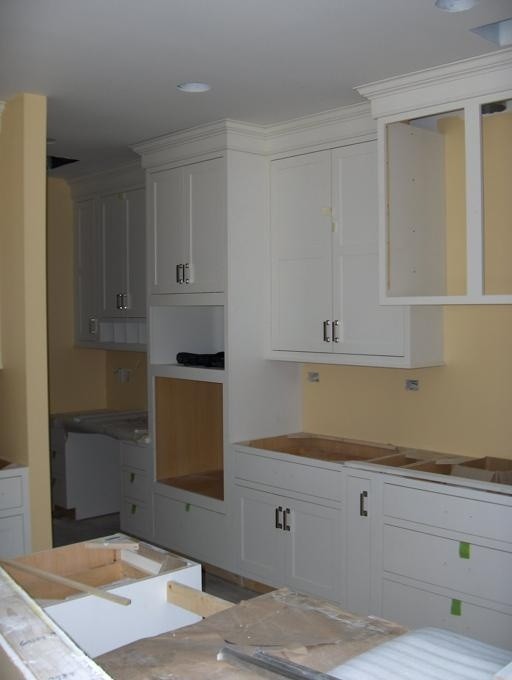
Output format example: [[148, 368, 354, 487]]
[[49, 423, 76, 515], [341, 471, 509, 654], [152, 379, 235, 565], [145, 157, 227, 367], [51, 406, 144, 437], [0, 466, 31, 559], [74, 198, 100, 348], [225, 448, 338, 603], [270, 142, 404, 359], [101, 185, 147, 353], [120, 443, 151, 537]]
